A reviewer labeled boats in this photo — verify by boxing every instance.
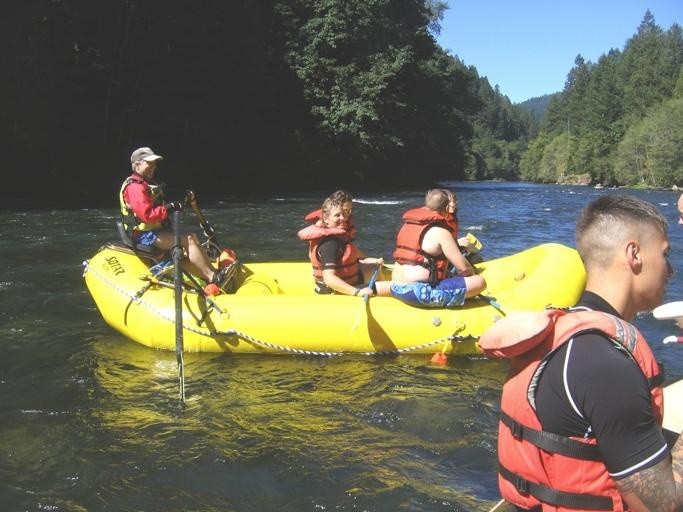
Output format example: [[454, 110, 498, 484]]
[[489, 379, 683, 511], [80, 240, 588, 360]]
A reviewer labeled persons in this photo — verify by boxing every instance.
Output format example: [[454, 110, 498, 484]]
[[442, 189, 481, 279], [661, 335, 683, 345], [377, 189, 486, 309], [310, 191, 384, 277], [675, 195, 683, 225], [311, 199, 372, 297], [495, 194, 683, 511], [116, 146, 242, 294]]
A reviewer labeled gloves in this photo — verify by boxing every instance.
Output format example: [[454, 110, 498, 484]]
[[171, 199, 187, 211]]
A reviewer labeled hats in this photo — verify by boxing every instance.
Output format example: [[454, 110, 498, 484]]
[[129, 146, 165, 163]]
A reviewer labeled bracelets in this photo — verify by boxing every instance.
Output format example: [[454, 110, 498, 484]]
[[353, 288, 360, 296]]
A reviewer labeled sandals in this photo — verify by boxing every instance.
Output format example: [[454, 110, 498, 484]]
[[209, 260, 239, 290]]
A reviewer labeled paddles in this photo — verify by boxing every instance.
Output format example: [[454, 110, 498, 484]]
[[449, 233, 483, 277]]
